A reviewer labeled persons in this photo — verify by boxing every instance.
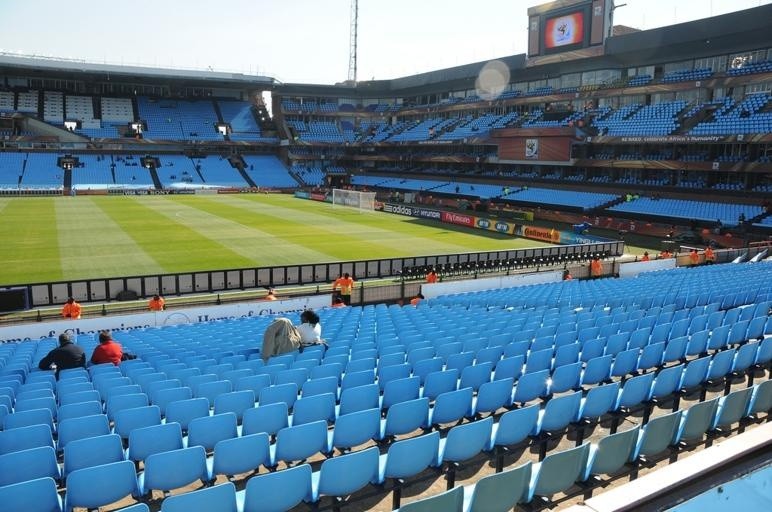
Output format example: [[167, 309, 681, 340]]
[[624, 190, 642, 204], [90, 330, 123, 368], [629, 216, 732, 269], [61, 296, 82, 320], [426, 269, 438, 283], [564, 270, 573, 281], [333, 272, 355, 307], [38, 333, 87, 371], [590, 255, 602, 279], [148, 294, 164, 312], [296, 310, 322, 345], [410, 293, 425, 305], [264, 291, 277, 300]]
